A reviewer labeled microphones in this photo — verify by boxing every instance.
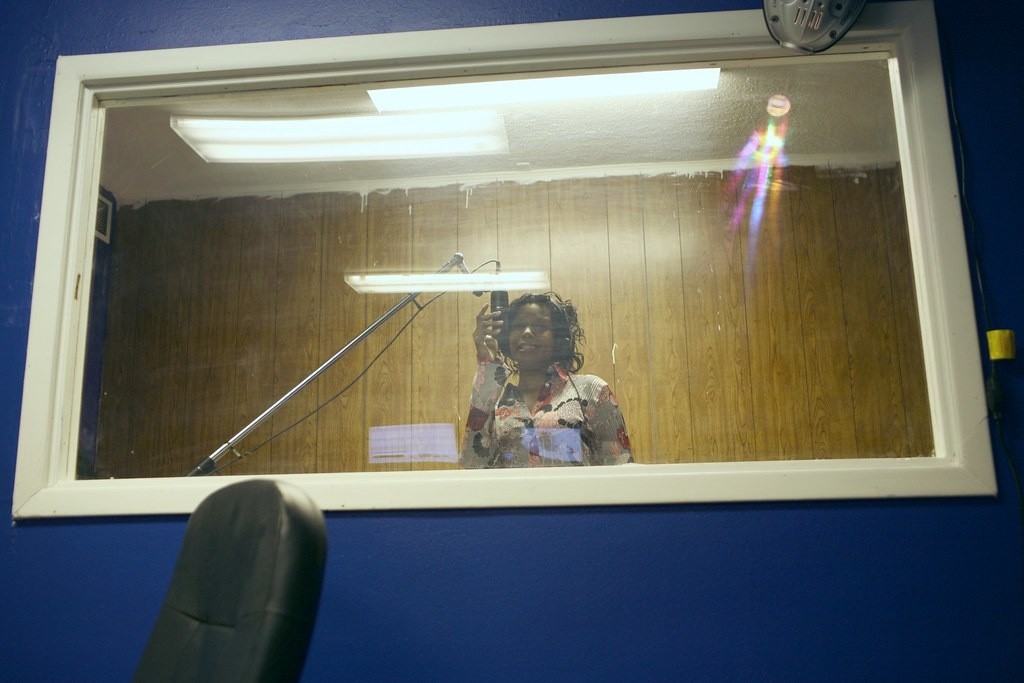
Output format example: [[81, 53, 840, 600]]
[[490, 261, 509, 333]]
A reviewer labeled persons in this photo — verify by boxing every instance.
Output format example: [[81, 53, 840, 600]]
[[459, 291, 635, 469]]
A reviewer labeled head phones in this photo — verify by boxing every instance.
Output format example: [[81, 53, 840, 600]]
[[567, 298, 575, 360]]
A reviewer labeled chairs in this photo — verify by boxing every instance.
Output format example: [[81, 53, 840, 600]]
[[135, 479, 327, 683]]
